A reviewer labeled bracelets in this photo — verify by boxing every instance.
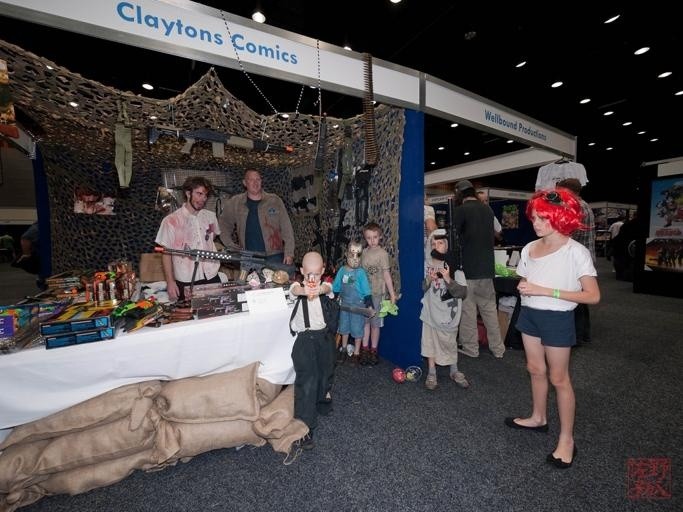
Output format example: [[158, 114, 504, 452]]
[[554, 289, 559, 298]]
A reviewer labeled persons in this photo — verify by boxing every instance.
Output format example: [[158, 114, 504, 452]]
[[154, 176, 221, 302], [333, 242, 376, 371], [556, 177, 597, 352], [503, 187, 601, 468], [420, 179, 507, 390], [605, 210, 638, 272], [288, 251, 339, 453], [218, 168, 296, 285], [361, 222, 397, 369]]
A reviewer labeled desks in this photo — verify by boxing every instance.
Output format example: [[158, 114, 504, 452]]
[[0, 287, 311, 512]]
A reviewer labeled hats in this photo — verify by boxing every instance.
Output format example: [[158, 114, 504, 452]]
[[454, 179, 474, 194]]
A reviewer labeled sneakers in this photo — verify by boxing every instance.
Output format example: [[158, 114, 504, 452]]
[[425, 372, 438, 391], [493, 351, 504, 358], [449, 369, 470, 388], [336, 348, 379, 369], [457, 343, 479, 358], [303, 438, 315, 450]]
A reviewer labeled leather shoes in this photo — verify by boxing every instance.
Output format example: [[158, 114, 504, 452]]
[[504, 416, 549, 434], [546, 440, 578, 470]]
